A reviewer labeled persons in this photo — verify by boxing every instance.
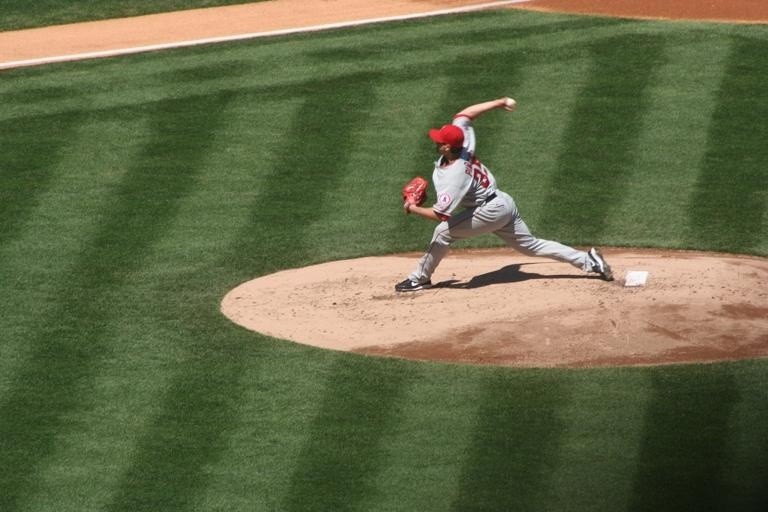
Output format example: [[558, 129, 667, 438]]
[[394, 96, 613, 291]]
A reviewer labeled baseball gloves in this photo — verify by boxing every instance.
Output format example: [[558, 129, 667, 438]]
[[402, 176, 429, 215]]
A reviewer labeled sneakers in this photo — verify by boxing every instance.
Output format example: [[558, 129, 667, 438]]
[[394, 275, 434, 293], [586, 246, 615, 283]]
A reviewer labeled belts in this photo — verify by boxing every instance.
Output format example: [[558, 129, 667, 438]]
[[482, 191, 499, 205]]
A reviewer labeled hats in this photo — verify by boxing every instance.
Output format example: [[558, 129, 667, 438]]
[[426, 123, 466, 149]]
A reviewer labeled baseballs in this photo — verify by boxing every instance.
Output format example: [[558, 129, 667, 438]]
[[506, 98, 518, 109]]
